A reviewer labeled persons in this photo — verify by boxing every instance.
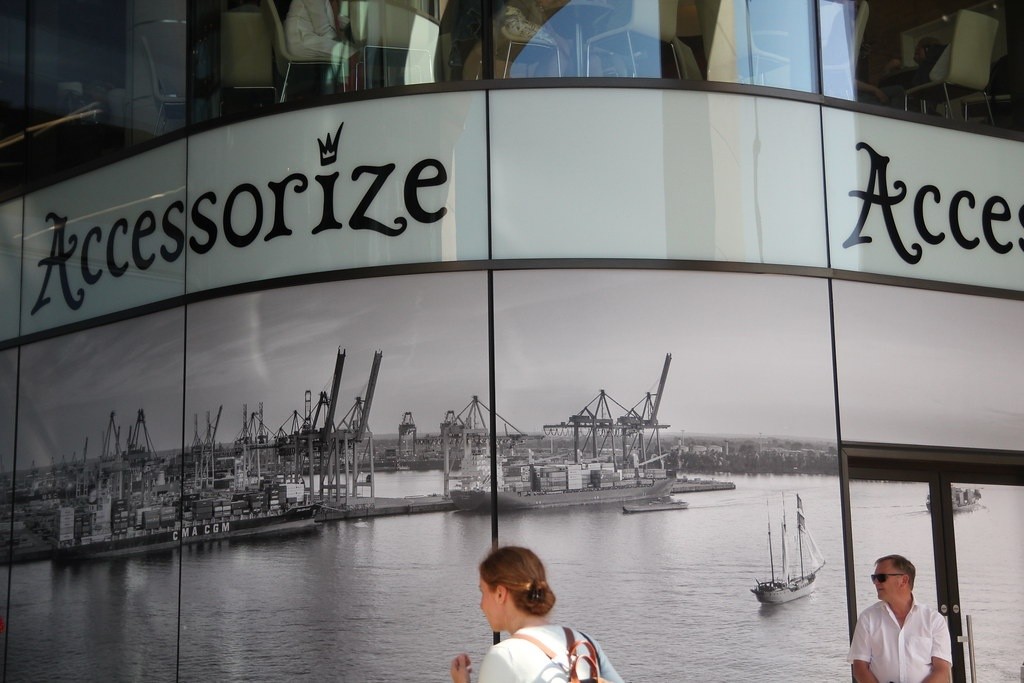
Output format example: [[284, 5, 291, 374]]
[[845, 554, 954, 682], [501, 0, 572, 73], [283, 0, 365, 96], [450, 545, 626, 683]]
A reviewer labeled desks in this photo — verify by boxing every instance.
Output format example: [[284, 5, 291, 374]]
[[544, 0, 613, 78]]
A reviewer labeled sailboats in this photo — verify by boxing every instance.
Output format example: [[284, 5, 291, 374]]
[[748, 490, 827, 605], [0, 347, 738, 578]]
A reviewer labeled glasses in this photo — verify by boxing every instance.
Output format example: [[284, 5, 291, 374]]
[[872, 574, 906, 583]]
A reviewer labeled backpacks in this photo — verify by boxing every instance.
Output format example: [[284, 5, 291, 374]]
[[511, 626, 610, 683]]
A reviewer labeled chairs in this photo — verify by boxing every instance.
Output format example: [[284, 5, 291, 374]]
[[586, 1, 683, 80], [350, 3, 431, 88], [735, 0, 792, 89], [135, 31, 187, 137], [259, 0, 348, 105], [215, 9, 279, 116], [810, 1, 871, 100], [904, 9, 1000, 124], [476, 0, 564, 80]]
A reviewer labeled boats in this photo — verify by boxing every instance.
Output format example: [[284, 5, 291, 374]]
[[925, 483, 982, 517]]
